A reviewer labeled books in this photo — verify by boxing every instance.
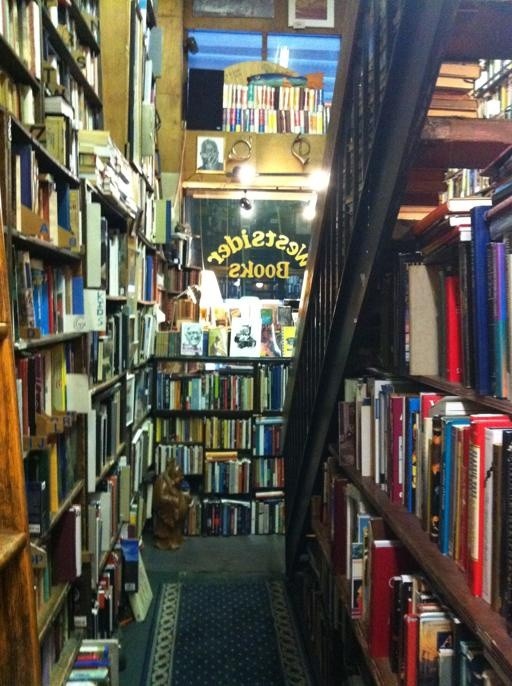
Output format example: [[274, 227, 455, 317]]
[[304, 145, 512, 686], [151, 292, 294, 537], [1, 0, 170, 686], [222, 84, 323, 135]]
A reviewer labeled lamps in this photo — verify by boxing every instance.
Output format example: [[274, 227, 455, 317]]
[[239, 197, 251, 212]]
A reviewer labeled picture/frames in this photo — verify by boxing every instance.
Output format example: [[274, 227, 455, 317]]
[[287, 0, 334, 29], [195, 135, 227, 175]]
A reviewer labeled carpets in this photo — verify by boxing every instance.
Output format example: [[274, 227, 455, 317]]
[[137, 573, 316, 685]]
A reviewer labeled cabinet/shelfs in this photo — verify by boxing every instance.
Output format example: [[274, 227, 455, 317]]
[[151, 356, 293, 537], [0, 0, 158, 686], [442, 59, 511, 198], [291, 359, 511, 681]]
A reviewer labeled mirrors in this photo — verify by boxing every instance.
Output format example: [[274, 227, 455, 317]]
[[182, 192, 312, 311]]
